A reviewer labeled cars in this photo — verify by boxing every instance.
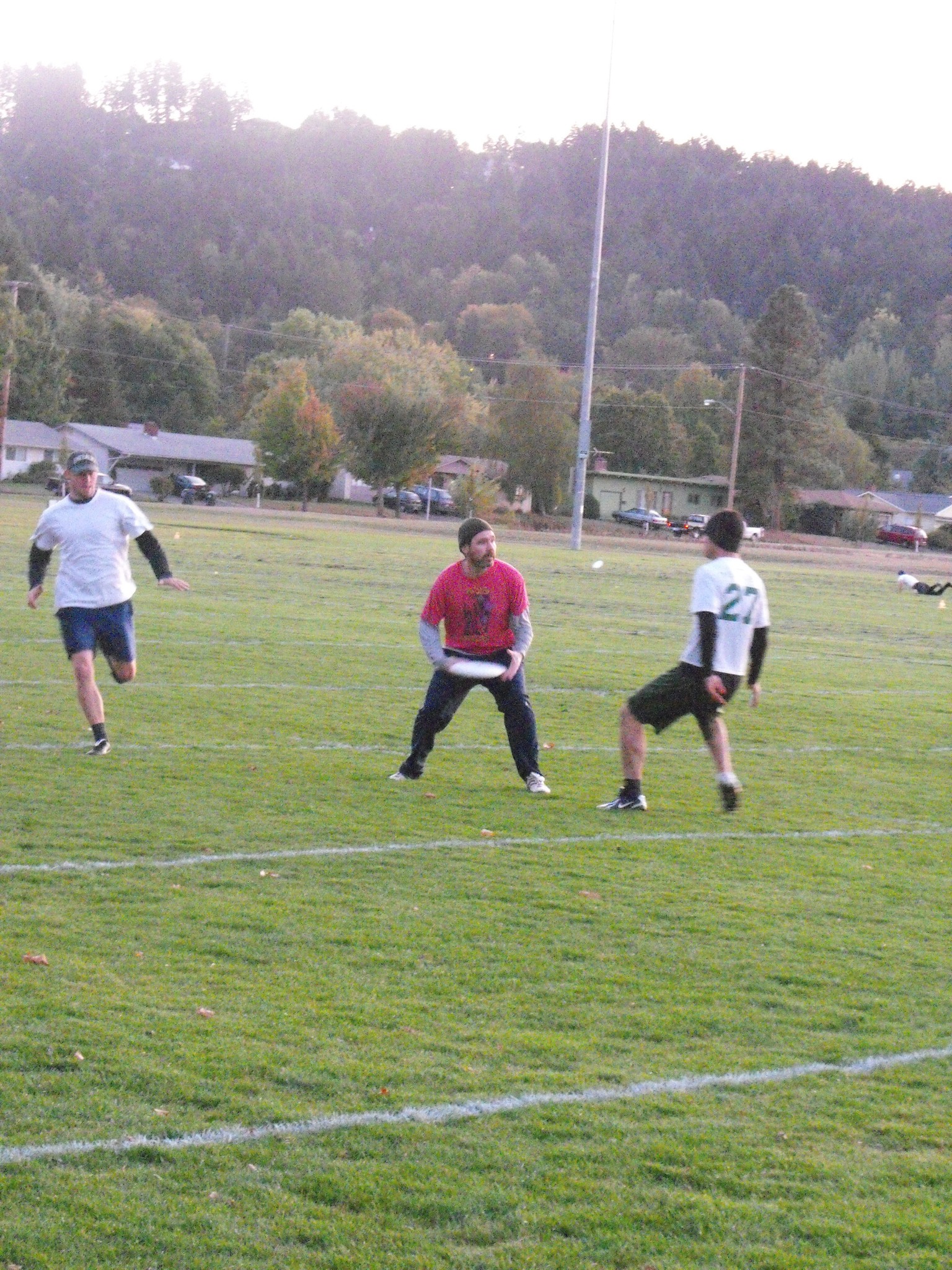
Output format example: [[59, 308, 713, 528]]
[[47, 474, 132, 497], [611, 506, 668, 530], [171, 476, 211, 498], [376, 486, 459, 517]]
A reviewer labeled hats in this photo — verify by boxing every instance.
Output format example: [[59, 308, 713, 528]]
[[66, 453, 98, 473], [705, 511, 743, 553], [459, 519, 492, 547]]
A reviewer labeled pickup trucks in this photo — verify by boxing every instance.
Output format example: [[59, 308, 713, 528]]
[[740, 522, 767, 541], [669, 515, 710, 538]]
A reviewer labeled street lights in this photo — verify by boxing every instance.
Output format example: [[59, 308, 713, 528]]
[[703, 397, 743, 513]]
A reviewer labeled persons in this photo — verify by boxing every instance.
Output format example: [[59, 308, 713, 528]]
[[595, 511, 771, 811], [897, 570, 952, 596], [25, 451, 189, 755], [388, 516, 551, 794]]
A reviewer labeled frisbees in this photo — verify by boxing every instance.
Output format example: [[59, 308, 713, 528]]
[[449, 660, 507, 679], [593, 561, 604, 568]]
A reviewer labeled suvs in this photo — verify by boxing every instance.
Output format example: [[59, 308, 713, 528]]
[[874, 526, 929, 547]]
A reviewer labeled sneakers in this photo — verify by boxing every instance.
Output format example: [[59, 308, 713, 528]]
[[388, 771, 407, 779], [86, 740, 111, 754], [525, 771, 550, 793], [718, 771, 742, 811], [597, 795, 647, 811]]
